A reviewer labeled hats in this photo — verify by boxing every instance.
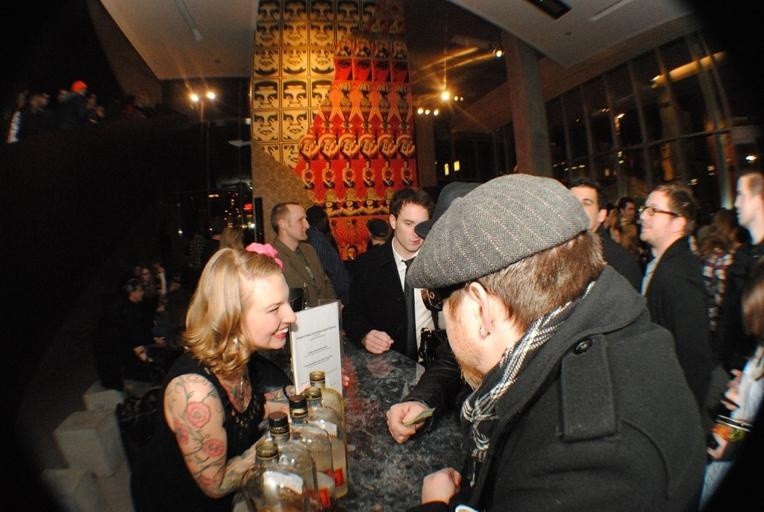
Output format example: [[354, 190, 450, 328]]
[[403, 173, 592, 290]]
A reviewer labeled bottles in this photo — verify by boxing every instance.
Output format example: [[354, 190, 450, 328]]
[[344, 219, 360, 260], [330, 221, 340, 245], [301, 159, 413, 187], [236, 369, 352, 512], [321, 188, 395, 217]]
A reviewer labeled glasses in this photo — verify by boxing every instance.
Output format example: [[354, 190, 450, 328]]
[[637, 205, 680, 220], [421, 279, 488, 312]]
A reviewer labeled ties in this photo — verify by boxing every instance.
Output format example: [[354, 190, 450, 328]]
[[401, 257, 419, 364]]
